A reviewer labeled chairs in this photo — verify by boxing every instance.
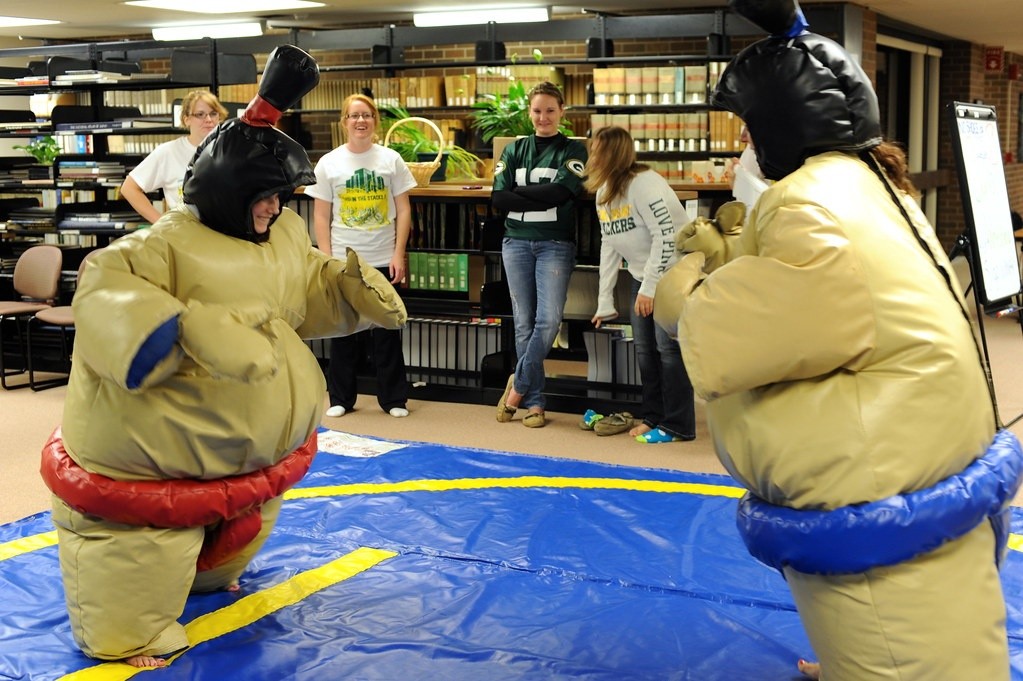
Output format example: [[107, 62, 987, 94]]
[[0, 247, 61, 390], [26, 248, 106, 391]]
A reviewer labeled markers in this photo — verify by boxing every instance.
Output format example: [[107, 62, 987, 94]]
[[996, 307, 1017, 316]]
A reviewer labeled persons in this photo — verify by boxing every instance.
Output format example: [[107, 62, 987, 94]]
[[304, 94, 418, 417], [41, 46, 407, 668], [491, 82, 589, 428], [654, 2, 1023, 681], [582, 126, 697, 444], [120, 91, 229, 224]]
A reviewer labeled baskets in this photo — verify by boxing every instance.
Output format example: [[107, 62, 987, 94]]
[[383, 117, 444, 188]]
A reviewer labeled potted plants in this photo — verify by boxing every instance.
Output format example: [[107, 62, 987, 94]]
[[376, 99, 484, 183]]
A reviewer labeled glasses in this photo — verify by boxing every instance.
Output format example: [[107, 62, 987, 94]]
[[345, 113, 376, 120], [187, 111, 218, 119]]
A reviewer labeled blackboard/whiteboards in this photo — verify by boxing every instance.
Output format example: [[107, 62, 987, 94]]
[[948, 100, 1021, 303]]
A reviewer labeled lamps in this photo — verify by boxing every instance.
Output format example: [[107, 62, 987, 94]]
[[151, 19, 266, 44], [413, 7, 553, 28]]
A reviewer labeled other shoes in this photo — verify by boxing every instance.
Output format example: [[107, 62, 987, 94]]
[[523, 411, 546, 427], [594, 412, 634, 436], [496, 373, 520, 423], [579, 422, 593, 429]]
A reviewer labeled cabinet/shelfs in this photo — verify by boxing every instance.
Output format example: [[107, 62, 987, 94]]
[[0, 7, 863, 419]]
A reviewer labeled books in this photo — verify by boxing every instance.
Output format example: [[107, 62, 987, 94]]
[[590, 61, 751, 221], [0, 118, 185, 153], [0, 161, 126, 209], [283, 197, 502, 372], [0, 213, 142, 248], [583, 328, 643, 386], [218, 64, 592, 149], [0, 69, 210, 115]]
